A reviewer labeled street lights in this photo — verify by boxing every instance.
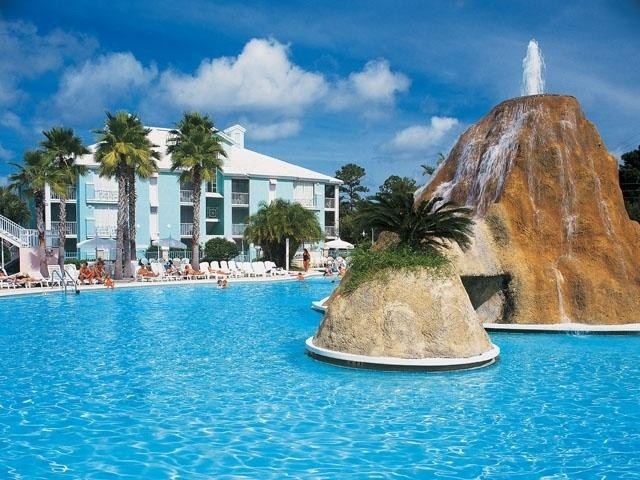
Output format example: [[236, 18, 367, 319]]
[[362, 227, 374, 246]]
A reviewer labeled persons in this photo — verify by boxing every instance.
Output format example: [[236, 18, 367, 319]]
[[297, 273, 304, 281], [302, 249, 311, 275], [0, 269, 43, 285], [323, 263, 343, 277], [77, 259, 233, 290]]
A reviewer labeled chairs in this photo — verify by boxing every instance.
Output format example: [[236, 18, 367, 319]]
[[129, 257, 288, 282], [0, 261, 106, 290]]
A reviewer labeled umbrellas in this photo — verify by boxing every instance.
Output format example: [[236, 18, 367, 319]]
[[151, 236, 187, 250], [76, 235, 115, 259], [104, 233, 116, 260], [323, 237, 354, 257]]
